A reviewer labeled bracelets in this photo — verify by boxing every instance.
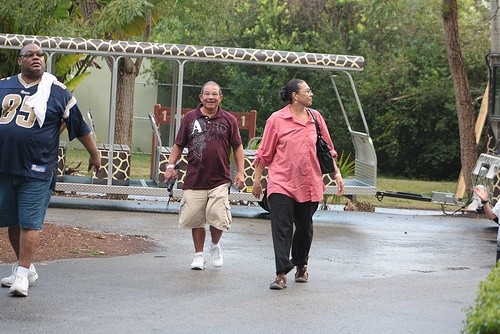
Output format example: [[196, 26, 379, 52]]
[[334, 173, 341, 180], [165, 164, 175, 169], [480, 198, 489, 204]]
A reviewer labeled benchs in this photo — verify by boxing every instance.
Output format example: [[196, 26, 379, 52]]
[[55, 141, 66, 182], [232, 137, 269, 193], [322, 164, 340, 186], [86, 111, 131, 185], [148, 111, 189, 188]]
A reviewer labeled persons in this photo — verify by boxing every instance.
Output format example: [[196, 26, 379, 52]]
[[251, 79, 344, 289], [0, 43, 102, 297], [472, 184, 500, 267], [163, 81, 246, 270]]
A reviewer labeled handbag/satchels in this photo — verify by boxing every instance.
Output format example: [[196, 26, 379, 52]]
[[308, 108, 335, 174]]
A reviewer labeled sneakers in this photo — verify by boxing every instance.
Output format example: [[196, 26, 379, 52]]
[[210, 243, 223, 266], [294, 264, 308, 281], [270, 273, 287, 289], [1, 263, 39, 287], [191, 253, 207, 269], [7, 271, 29, 297]]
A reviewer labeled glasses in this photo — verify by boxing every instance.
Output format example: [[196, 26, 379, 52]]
[[19, 53, 44, 60], [296, 90, 312, 94]]
